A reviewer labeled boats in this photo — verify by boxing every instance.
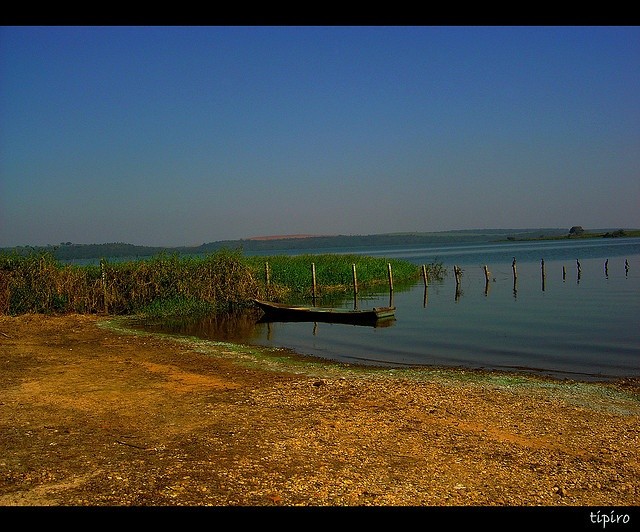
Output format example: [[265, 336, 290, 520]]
[[255, 314, 397, 328], [252, 297, 396, 318]]
